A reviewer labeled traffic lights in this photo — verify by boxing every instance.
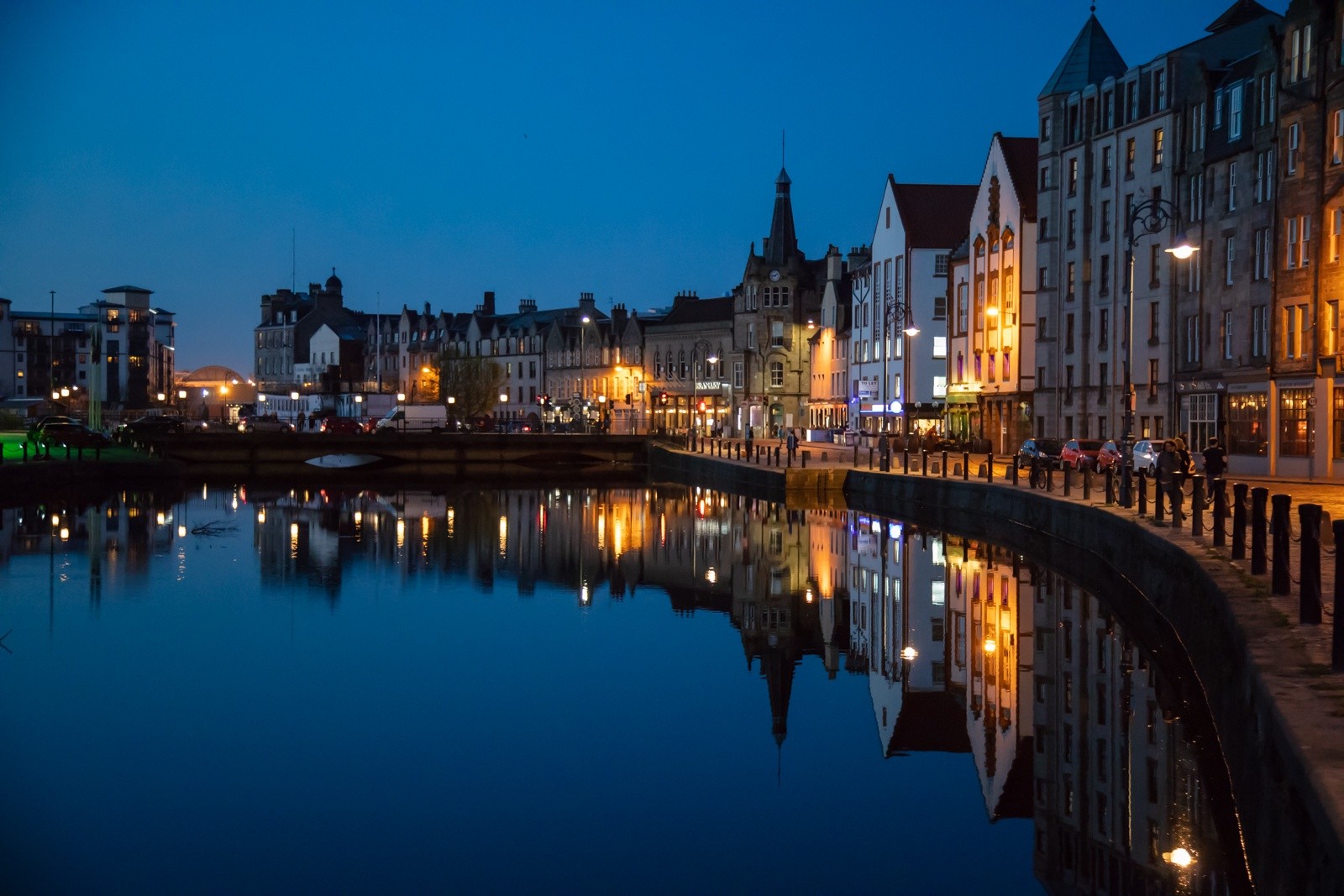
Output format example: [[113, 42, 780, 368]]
[[544, 394, 552, 408], [660, 392, 668, 406], [536, 394, 541, 406]]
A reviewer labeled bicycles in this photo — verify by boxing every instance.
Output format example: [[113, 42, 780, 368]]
[[1028, 458, 1046, 488]]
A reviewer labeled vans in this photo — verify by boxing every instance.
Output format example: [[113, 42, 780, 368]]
[[376, 405, 448, 436]]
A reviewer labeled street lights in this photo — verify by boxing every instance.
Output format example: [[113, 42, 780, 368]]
[[48, 290, 57, 415], [1118, 198, 1204, 509], [878, 302, 920, 472], [580, 317, 591, 404], [224, 371, 239, 429], [691, 357, 719, 452]]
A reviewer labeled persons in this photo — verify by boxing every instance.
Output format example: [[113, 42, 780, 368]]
[[263, 408, 316, 434], [774, 424, 784, 449], [749, 426, 755, 451], [717, 420, 723, 438], [786, 430, 799, 460], [1202, 437, 1228, 502], [1155, 432, 1191, 520]]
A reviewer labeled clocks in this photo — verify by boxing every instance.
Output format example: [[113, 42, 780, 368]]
[[769, 270, 781, 282]]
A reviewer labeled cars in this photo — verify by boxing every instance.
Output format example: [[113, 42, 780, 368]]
[[116, 416, 211, 438], [1017, 437, 1061, 470], [238, 415, 295, 434], [1059, 438, 1107, 472], [458, 416, 540, 434], [1096, 439, 1196, 477], [320, 416, 384, 435], [36, 415, 112, 448]]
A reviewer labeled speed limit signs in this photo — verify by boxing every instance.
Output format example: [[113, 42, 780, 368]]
[[1308, 397, 1317, 406]]
